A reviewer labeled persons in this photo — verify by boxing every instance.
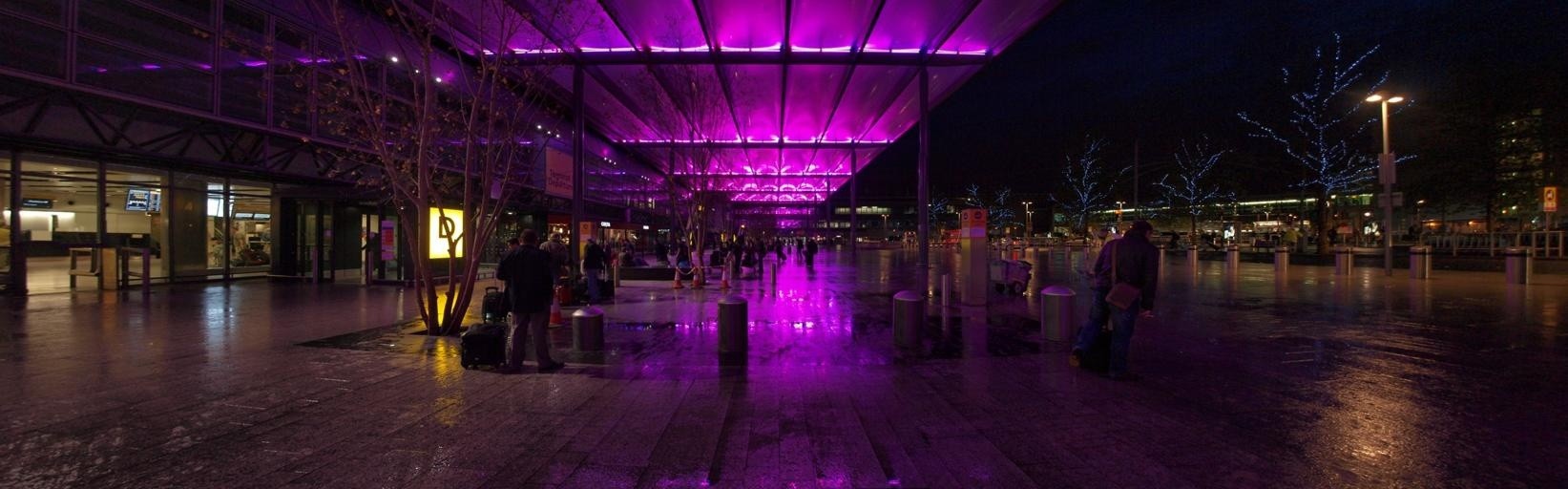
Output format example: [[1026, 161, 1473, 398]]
[[722, 233, 822, 274], [650, 236, 691, 267], [1071, 220, 1159, 380], [496, 232, 636, 372]]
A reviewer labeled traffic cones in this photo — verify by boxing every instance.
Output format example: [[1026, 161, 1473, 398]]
[[547, 290, 565, 329], [691, 267, 704, 289], [673, 267, 683, 289], [721, 265, 731, 288]]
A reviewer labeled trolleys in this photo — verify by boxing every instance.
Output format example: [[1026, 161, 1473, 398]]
[[992, 244, 1025, 296]]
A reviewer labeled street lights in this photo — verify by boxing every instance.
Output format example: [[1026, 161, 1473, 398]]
[[1363, 92, 1413, 271], [640, 224, 649, 254], [599, 220, 610, 246], [880, 213, 890, 241], [1115, 199, 1126, 236], [1021, 200, 1033, 235]]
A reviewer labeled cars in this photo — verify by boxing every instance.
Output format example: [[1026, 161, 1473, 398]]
[[988, 235, 1098, 252]]
